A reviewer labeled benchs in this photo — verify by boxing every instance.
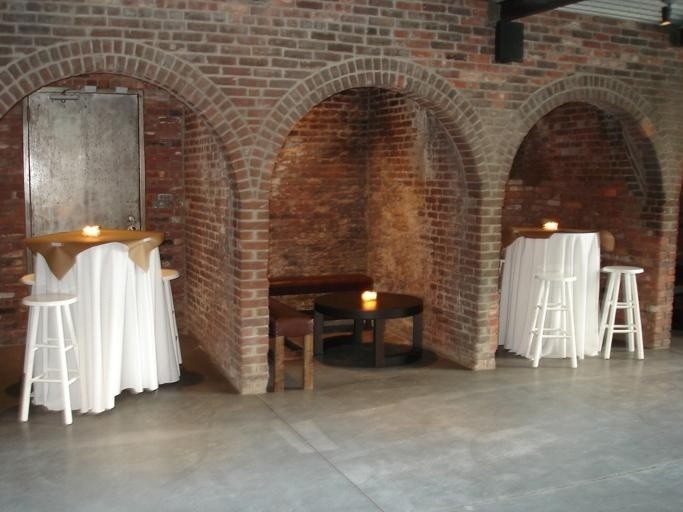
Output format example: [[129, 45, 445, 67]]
[[269, 274, 373, 392]]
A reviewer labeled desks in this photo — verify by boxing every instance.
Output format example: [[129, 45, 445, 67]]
[[313, 291, 422, 367], [20, 229, 179, 414], [498, 227, 601, 360]]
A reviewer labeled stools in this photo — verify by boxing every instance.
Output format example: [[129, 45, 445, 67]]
[[160, 269, 182, 364], [598, 266, 644, 359], [525, 273, 578, 368], [20, 273, 35, 285], [19, 294, 78, 425]]
